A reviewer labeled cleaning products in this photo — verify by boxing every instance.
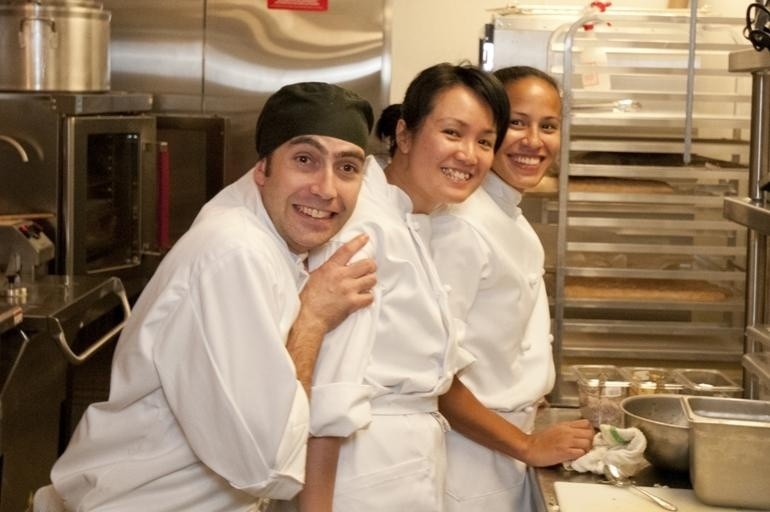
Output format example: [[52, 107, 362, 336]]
[[581, 2, 612, 92]]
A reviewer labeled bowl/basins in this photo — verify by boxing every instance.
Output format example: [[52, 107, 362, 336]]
[[617, 393, 689, 474]]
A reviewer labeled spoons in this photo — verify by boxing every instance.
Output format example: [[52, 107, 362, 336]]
[[601, 463, 681, 512]]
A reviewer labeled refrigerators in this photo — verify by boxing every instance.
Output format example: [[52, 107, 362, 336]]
[[114, 0, 394, 300]]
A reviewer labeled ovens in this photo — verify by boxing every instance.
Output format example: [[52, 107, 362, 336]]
[[0, 92, 176, 286]]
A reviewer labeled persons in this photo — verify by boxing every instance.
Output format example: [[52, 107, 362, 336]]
[[433, 66, 594, 512], [284, 61, 508, 512], [26, 82, 378, 512]]
